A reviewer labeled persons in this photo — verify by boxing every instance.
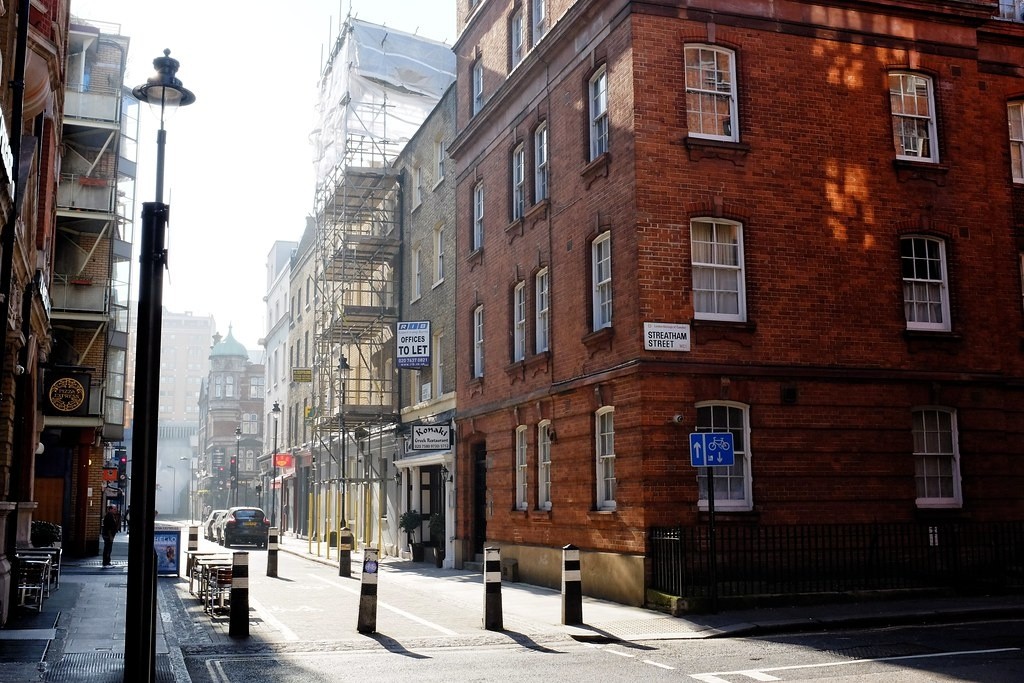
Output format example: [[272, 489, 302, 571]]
[[164, 546, 175, 564], [102, 505, 118, 568], [125, 504, 130, 534]]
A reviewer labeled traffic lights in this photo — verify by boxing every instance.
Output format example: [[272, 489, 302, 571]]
[[230, 456, 237, 489], [218, 466, 226, 492], [256, 485, 261, 493], [119, 454, 126, 474]]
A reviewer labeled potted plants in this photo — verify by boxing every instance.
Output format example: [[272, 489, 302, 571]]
[[78, 170, 108, 187], [429, 513, 446, 568], [70, 273, 94, 284], [398, 510, 425, 562]]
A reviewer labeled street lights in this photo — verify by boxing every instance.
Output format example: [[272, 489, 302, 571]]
[[333, 354, 354, 528], [167, 465, 175, 514], [123, 48, 195, 683], [180, 457, 193, 524], [271, 401, 281, 527], [234, 425, 241, 507]]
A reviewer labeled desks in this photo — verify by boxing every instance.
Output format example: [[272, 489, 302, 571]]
[[184, 550, 233, 612], [11, 560, 51, 612], [18, 549, 63, 589]]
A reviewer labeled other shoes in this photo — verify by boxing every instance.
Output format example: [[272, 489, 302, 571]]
[[103, 561, 111, 566]]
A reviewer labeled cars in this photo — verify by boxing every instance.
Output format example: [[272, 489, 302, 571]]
[[203, 510, 229, 542]]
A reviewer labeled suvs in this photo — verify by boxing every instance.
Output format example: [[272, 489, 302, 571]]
[[216, 507, 270, 550]]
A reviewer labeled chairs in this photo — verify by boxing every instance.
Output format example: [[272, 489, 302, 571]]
[[209, 568, 232, 621]]
